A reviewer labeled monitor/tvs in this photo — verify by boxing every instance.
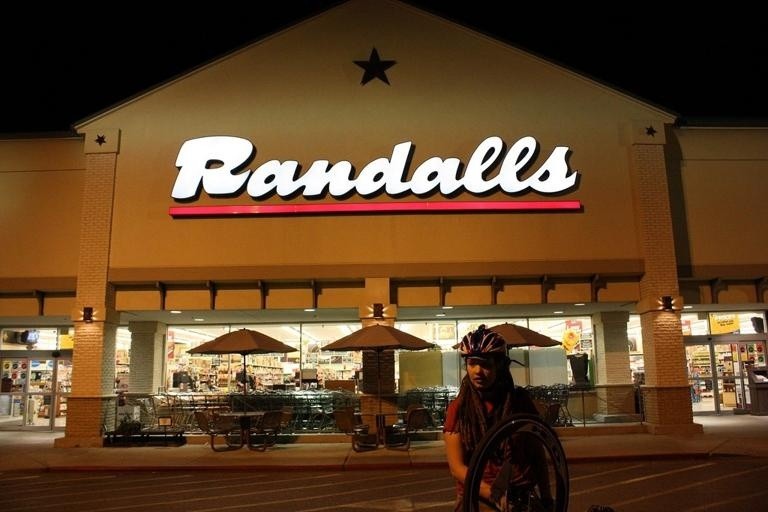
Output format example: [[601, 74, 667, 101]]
[[21, 330, 38, 345]]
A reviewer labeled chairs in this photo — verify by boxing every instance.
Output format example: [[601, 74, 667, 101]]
[[332, 403, 428, 453], [193, 405, 295, 452]]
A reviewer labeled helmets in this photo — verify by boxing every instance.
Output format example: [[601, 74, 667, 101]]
[[457, 329, 507, 361]]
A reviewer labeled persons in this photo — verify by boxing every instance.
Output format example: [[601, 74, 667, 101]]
[[284, 365, 300, 384], [1, 373, 13, 392], [442, 324, 538, 512], [231, 363, 264, 393]]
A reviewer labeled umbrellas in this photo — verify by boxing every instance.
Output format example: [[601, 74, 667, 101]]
[[320, 324, 434, 429], [186, 327, 297, 413], [452, 321, 558, 356]]
